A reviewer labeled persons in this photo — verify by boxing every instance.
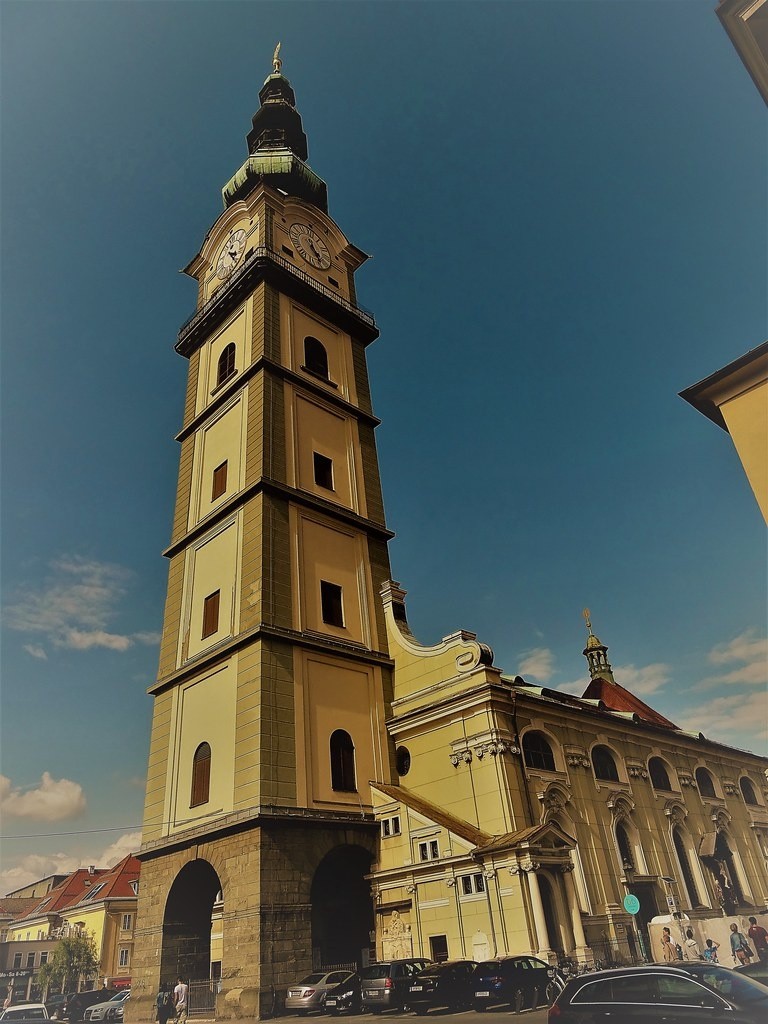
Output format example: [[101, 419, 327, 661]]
[[682, 929, 707, 962], [728, 923, 752, 966], [662, 934, 680, 962], [547, 956, 576, 1008], [659, 926, 683, 960], [746, 916, 768, 961], [703, 939, 721, 965], [155, 982, 173, 1024], [173, 976, 189, 1024]]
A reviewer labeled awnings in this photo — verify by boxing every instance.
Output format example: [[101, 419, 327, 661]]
[[110, 979, 132, 989]]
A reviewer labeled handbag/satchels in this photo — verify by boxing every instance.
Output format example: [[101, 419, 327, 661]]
[[699, 955, 704, 960], [738, 933, 754, 957]]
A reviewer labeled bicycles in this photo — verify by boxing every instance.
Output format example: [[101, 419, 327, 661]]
[[550, 956, 649, 1008]]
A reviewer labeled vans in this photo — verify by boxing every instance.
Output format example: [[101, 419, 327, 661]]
[[359, 958, 434, 1014]]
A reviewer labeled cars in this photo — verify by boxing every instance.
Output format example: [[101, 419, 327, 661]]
[[547, 963, 768, 1024], [283, 970, 355, 1015], [463, 955, 552, 1013], [403, 960, 480, 1015], [320, 978, 362, 1016], [0, 988, 131, 1024]]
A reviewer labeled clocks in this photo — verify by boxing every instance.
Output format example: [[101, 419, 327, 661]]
[[216, 228, 247, 280], [288, 223, 332, 271]]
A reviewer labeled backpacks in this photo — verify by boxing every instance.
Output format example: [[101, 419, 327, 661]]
[[163, 992, 172, 1006]]
